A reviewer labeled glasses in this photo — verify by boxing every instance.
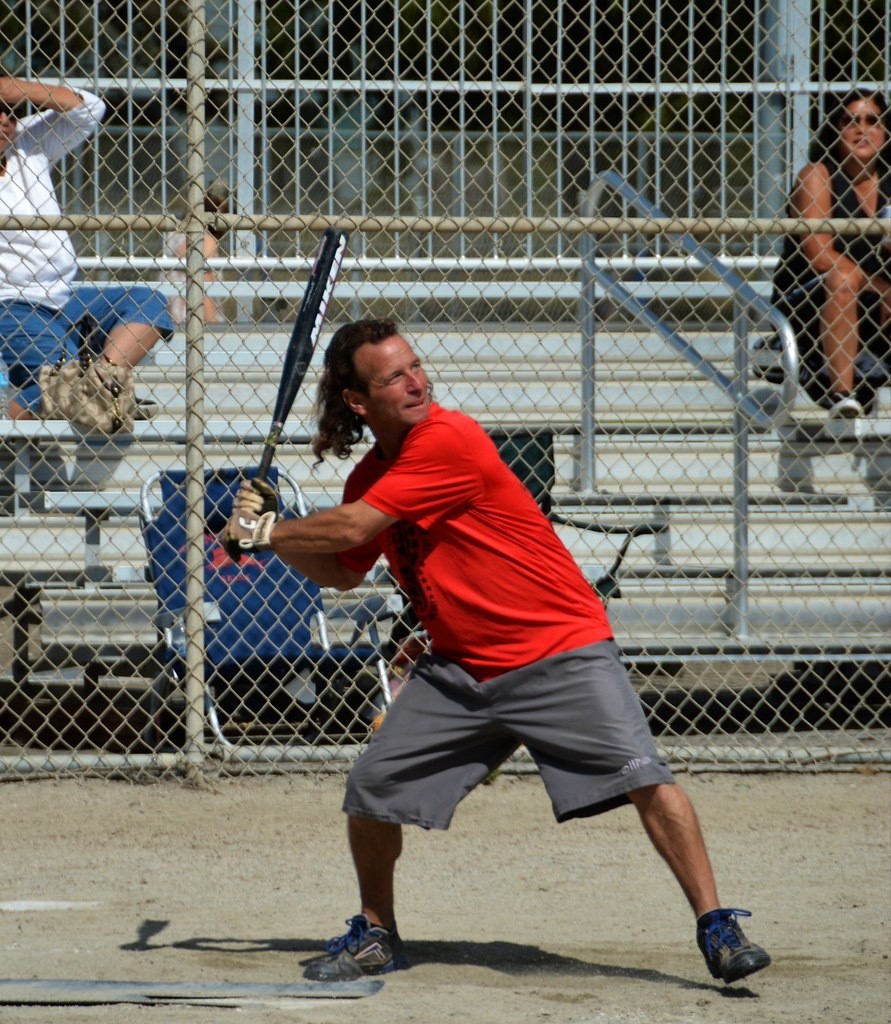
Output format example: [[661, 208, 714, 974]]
[[840, 114, 884, 126]]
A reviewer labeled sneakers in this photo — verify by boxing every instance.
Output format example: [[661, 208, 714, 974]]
[[696, 908, 771, 983], [303, 915, 408, 982]]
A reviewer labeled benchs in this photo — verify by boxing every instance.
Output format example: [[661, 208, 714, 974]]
[[0, 280, 891, 733]]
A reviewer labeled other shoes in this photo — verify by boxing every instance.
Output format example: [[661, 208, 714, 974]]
[[133, 396, 157, 420], [829, 391, 861, 419]]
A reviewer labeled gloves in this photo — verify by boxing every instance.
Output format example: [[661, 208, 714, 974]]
[[222, 509, 280, 563], [230, 478, 278, 523]]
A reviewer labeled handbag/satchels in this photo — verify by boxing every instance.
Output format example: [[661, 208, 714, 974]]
[[39, 317, 133, 434]]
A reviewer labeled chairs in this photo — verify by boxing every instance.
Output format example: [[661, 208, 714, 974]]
[[141, 467, 392, 747], [391, 428, 667, 678]]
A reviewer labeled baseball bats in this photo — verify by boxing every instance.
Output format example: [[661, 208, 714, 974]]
[[224, 226, 352, 563]]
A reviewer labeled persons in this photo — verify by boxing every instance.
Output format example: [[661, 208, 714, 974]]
[[162, 179, 235, 323], [221, 320, 770, 982], [771, 90, 891, 417], [0, 75, 174, 435]]
